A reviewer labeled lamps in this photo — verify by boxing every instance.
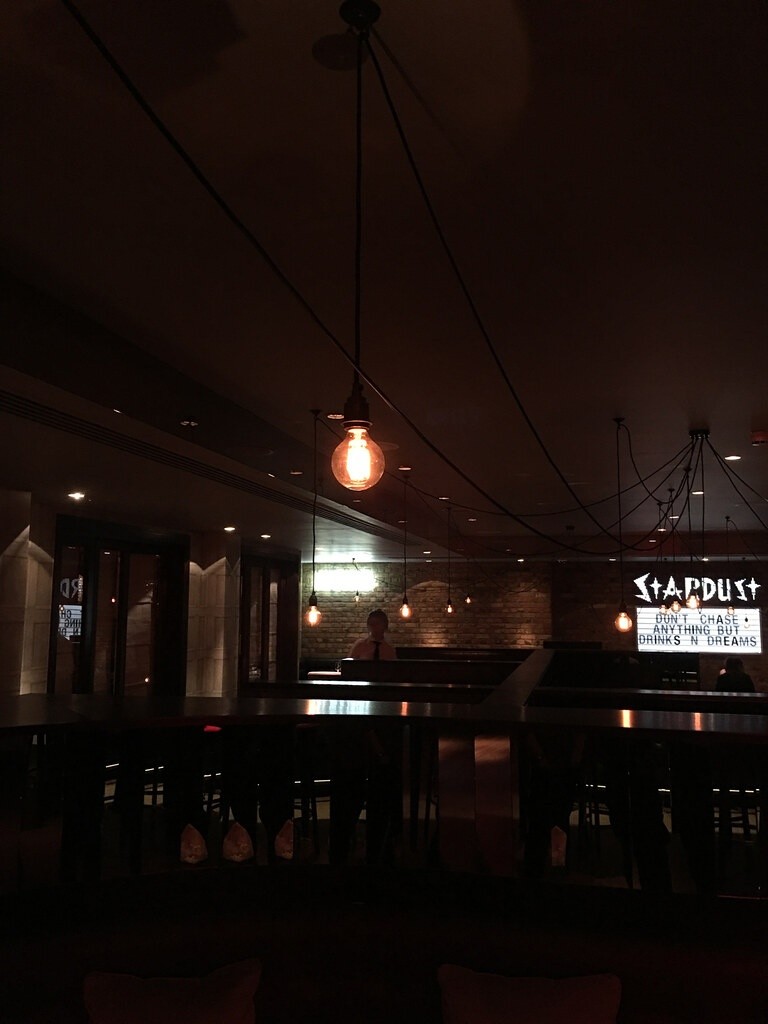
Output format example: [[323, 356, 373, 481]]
[[330, 39, 386, 489], [398, 474, 413, 619], [684, 467, 699, 608], [667, 488, 681, 614], [463, 558, 472, 604], [657, 500, 668, 615], [355, 591, 360, 601], [727, 517, 738, 614], [614, 420, 633, 633], [303, 407, 324, 627], [443, 508, 455, 616]]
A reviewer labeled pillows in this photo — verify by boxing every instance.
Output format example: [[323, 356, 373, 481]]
[[435, 962, 623, 1023], [79, 958, 266, 1023]]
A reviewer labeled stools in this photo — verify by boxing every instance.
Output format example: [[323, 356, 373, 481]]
[[22, 709, 768, 897]]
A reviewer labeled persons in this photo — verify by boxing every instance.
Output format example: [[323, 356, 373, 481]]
[[349, 609, 396, 659], [715, 656, 755, 692]]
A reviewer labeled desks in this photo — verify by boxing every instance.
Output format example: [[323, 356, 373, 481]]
[[392, 642, 546, 655], [531, 683, 768, 708], [2, 694, 480, 874], [559, 638, 700, 660], [355, 655, 527, 674], [299, 675, 500, 705], [483, 702, 767, 890]]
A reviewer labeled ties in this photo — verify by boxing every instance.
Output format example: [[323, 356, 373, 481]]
[[371, 640, 382, 661]]
